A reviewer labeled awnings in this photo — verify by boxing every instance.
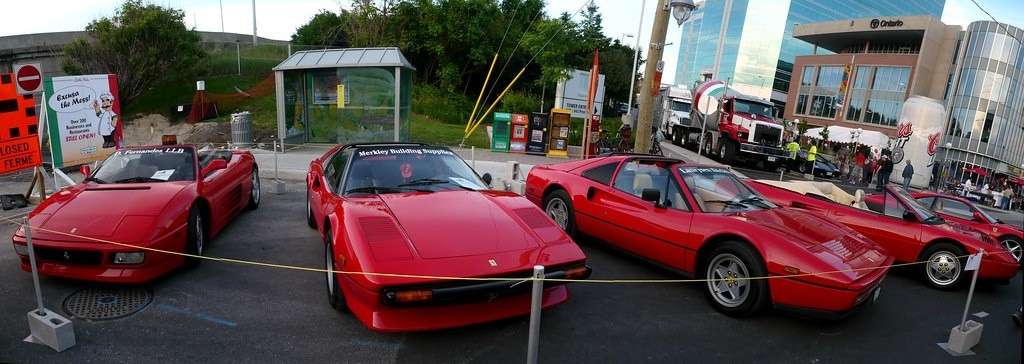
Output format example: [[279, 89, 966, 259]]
[[965, 167, 990, 177], [792, 126, 896, 150], [1007, 176, 1024, 185]]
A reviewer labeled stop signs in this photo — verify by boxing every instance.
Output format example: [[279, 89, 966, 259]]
[[12, 62, 44, 95]]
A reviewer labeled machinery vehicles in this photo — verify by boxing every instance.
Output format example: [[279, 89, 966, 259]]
[[671, 80, 790, 173], [652, 82, 693, 141]]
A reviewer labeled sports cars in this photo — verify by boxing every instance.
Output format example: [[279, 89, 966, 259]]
[[306, 142, 593, 331], [12, 144, 262, 284], [863, 191, 1024, 269], [525, 154, 896, 319], [740, 181, 1020, 292]]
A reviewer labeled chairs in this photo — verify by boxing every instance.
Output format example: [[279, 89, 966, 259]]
[[676, 176, 709, 213], [852, 188, 869, 211], [936, 201, 946, 214], [124, 158, 146, 179], [345, 160, 376, 195], [632, 174, 661, 207], [179, 154, 202, 179]]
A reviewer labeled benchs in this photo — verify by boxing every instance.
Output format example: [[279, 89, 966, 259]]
[[360, 116, 393, 129]]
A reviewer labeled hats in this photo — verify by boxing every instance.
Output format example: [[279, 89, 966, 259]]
[[882, 155, 888, 158]]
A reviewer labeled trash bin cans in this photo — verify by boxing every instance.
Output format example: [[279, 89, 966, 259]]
[[231, 111, 252, 145]]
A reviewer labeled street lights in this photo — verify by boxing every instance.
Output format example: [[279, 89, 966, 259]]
[[634, 0, 696, 154]]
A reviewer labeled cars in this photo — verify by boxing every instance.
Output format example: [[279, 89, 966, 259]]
[[788, 150, 841, 178]]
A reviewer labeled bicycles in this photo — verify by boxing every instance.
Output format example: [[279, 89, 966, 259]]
[[650, 125, 664, 156], [594, 128, 634, 158]]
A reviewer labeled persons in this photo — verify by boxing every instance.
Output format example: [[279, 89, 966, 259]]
[[787, 139, 804, 178], [783, 128, 831, 152], [806, 139, 817, 175], [902, 160, 914, 191], [947, 175, 1024, 210], [836, 144, 894, 192]]
